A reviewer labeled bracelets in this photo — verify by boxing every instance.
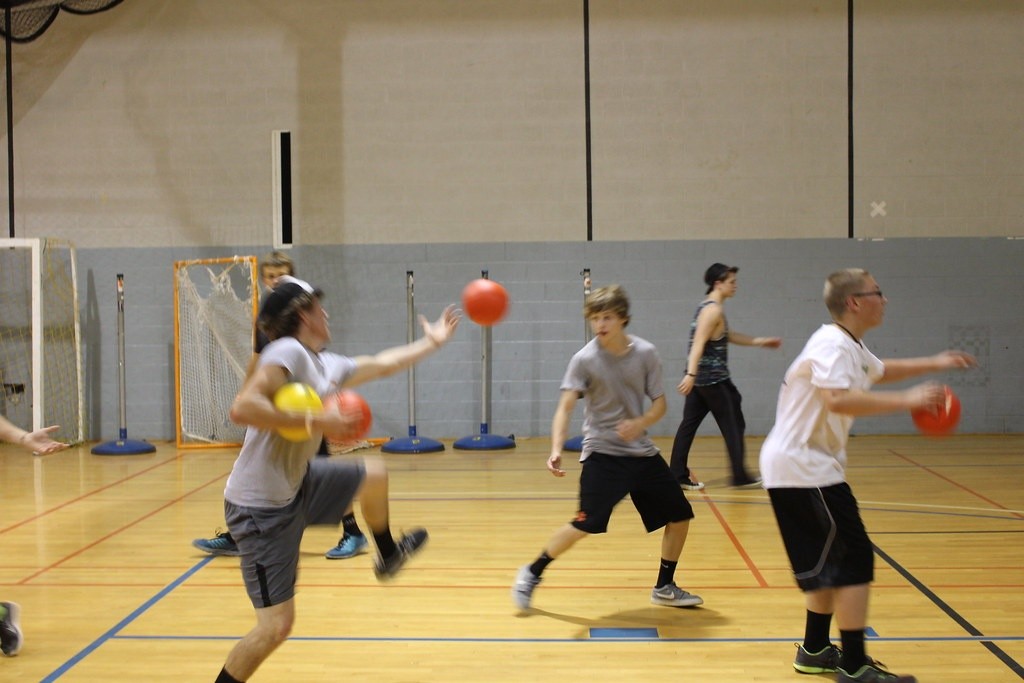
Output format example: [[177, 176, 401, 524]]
[[686, 373, 696, 376], [21, 433, 28, 445]]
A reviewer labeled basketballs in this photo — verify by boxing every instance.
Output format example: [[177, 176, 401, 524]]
[[324, 390, 372, 443], [911, 383, 961, 434], [464, 279, 509, 326]]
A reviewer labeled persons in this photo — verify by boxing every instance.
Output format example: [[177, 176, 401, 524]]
[[757, 268, 973, 683], [0, 414, 70, 656], [669, 263, 783, 488], [512, 284, 705, 612], [193, 254, 368, 560], [213, 277, 462, 683]]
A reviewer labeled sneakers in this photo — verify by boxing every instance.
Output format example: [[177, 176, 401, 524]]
[[793, 640, 873, 674], [650, 582, 703, 607], [326, 531, 369, 558], [0, 601, 23, 656], [837, 653, 917, 683], [513, 564, 541, 610], [192, 527, 241, 556], [680, 478, 705, 489], [730, 477, 763, 489]]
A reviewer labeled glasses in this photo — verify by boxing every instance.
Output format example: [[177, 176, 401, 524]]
[[853, 288, 883, 298]]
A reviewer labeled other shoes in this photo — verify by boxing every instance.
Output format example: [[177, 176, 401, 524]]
[[374, 528, 427, 580]]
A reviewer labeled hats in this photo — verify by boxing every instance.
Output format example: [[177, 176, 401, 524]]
[[704, 262, 729, 294], [254, 274, 313, 353]]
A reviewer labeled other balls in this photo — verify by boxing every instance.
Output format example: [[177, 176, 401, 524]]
[[272, 380, 324, 442]]
